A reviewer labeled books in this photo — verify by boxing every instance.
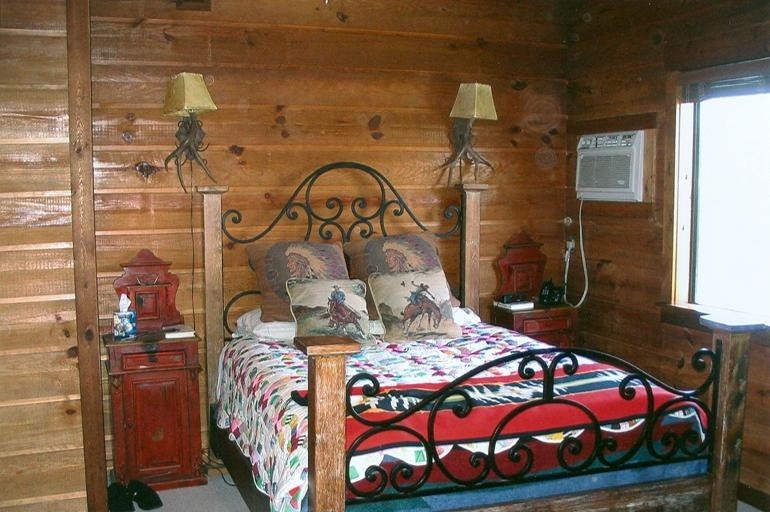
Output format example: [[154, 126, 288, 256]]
[[493, 298, 535, 312], [162, 323, 196, 340]]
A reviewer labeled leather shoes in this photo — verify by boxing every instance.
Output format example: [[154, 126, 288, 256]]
[[107, 478, 163, 512]]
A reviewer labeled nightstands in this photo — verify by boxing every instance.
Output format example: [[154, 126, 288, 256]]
[[488, 290, 577, 352], [104, 327, 203, 495]]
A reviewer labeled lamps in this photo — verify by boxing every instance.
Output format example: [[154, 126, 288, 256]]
[[434, 82, 502, 189], [162, 69, 218, 193]]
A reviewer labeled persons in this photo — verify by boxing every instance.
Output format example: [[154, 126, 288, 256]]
[[329, 284, 351, 323], [409, 280, 435, 315]]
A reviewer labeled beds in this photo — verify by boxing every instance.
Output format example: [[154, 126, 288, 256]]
[[196, 160, 770, 511]]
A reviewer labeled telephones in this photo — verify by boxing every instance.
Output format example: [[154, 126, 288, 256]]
[[502, 292, 529, 304]]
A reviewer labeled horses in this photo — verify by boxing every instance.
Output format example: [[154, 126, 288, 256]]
[[400, 297, 442, 334], [327, 296, 365, 340]]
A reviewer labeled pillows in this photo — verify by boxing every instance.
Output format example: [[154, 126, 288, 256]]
[[231, 230, 469, 349]]
